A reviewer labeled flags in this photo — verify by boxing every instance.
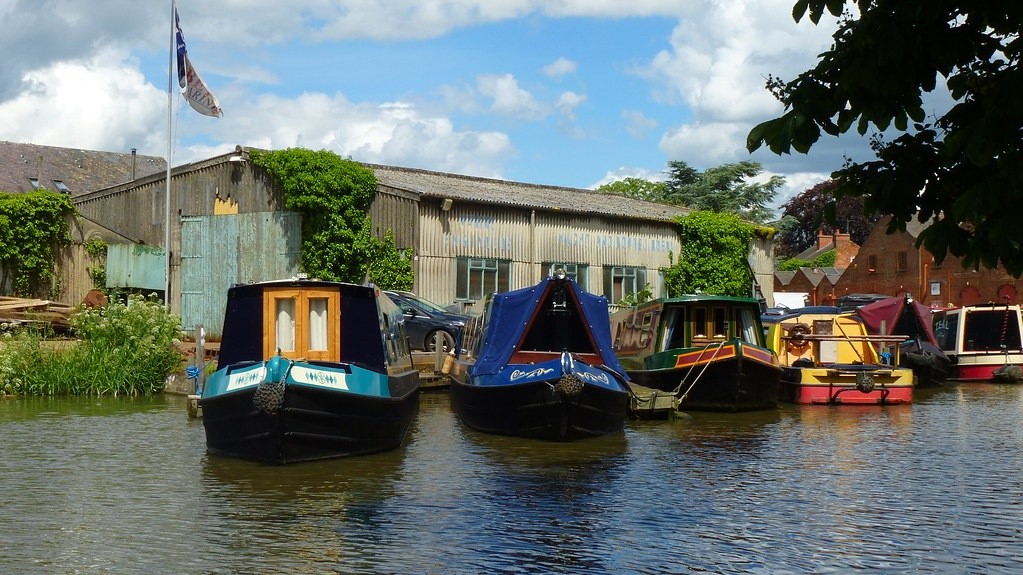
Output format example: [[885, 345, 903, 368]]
[[174, 7, 223, 118]]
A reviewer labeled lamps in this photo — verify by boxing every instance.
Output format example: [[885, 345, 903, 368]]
[[440, 198, 453, 211], [229, 156, 246, 168]]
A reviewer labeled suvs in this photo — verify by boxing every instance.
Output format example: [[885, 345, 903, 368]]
[[380, 289, 476, 352]]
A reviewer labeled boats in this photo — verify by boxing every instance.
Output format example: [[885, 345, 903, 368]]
[[932, 300, 1023, 379], [755, 302, 918, 404], [836, 295, 952, 392], [198, 278, 420, 465], [612, 291, 785, 414], [445, 266, 631, 442]]
